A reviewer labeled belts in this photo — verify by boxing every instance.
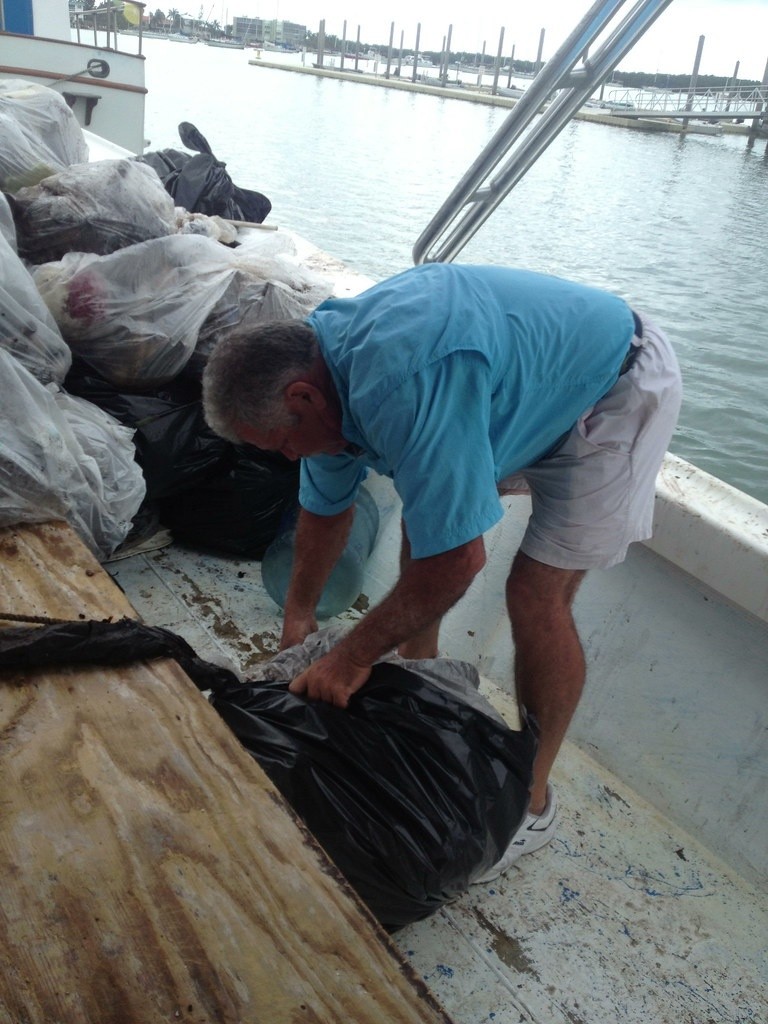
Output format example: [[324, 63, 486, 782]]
[[619, 310, 642, 375]]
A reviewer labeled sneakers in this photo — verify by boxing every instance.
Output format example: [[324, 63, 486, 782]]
[[471, 781, 558, 883]]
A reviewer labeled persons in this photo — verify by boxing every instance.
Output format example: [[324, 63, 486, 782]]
[[204, 264, 682, 885]]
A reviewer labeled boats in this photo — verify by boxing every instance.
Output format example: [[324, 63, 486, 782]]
[[248, 0, 496, 74], [0, 0, 150, 163], [204, 37, 245, 50], [642, 69, 674, 95], [120, 23, 170, 39], [169, 34, 200, 44]]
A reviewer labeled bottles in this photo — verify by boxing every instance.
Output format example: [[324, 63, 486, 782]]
[[261, 481, 378, 616]]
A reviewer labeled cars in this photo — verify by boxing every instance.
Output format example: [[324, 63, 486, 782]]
[[502, 65, 516, 74]]
